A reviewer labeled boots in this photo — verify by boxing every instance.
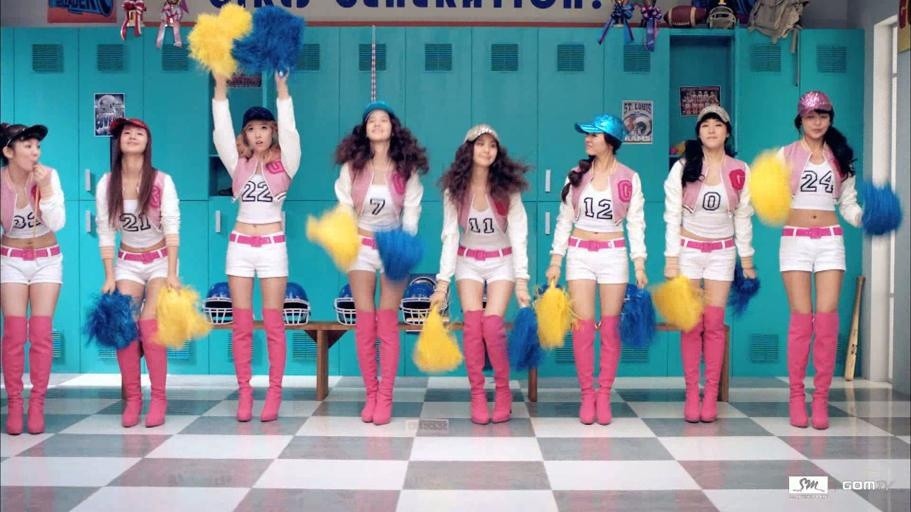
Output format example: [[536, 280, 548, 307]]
[[787, 312, 814, 428], [231, 306, 253, 423], [811, 308, 839, 429], [680, 313, 704, 423], [462, 310, 491, 425], [261, 307, 286, 422], [356, 311, 379, 423], [1, 315, 28, 436], [700, 305, 726, 422], [138, 321, 168, 427], [27, 317, 53, 435], [596, 314, 621, 425], [373, 310, 400, 425], [482, 315, 514, 422], [116, 324, 144, 426], [570, 317, 599, 425]]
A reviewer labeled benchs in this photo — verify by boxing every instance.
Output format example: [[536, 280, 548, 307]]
[[214, 321, 729, 402]]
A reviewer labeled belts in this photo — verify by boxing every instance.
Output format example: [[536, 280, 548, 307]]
[[361, 238, 383, 250], [117, 248, 167, 265], [568, 238, 626, 252], [680, 239, 735, 253], [783, 227, 843, 239], [229, 233, 286, 247], [0, 245, 61, 260], [457, 246, 512, 261]]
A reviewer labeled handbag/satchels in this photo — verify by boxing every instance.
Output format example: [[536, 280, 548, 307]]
[[745, 0, 803, 39]]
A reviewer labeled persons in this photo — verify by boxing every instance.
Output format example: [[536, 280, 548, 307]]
[[0, 121, 65, 432], [94, 115, 180, 428], [212, 65, 302, 423], [662, 105, 759, 426], [757, 87, 866, 429], [544, 112, 648, 427], [427, 122, 532, 426], [332, 98, 430, 425]]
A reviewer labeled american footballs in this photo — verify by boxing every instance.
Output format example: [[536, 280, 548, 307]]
[[663, 5, 706, 27]]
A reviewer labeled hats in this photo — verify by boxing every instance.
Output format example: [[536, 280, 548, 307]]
[[463, 123, 500, 147], [109, 117, 147, 136], [2, 121, 48, 157], [697, 105, 732, 124], [241, 106, 275, 129], [798, 90, 832, 116], [575, 113, 627, 143], [362, 102, 395, 119]]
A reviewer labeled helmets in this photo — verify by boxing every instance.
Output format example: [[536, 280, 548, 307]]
[[704, 2, 738, 30], [334, 284, 357, 328], [400, 277, 450, 328], [282, 281, 311, 328], [202, 282, 233, 326]]
